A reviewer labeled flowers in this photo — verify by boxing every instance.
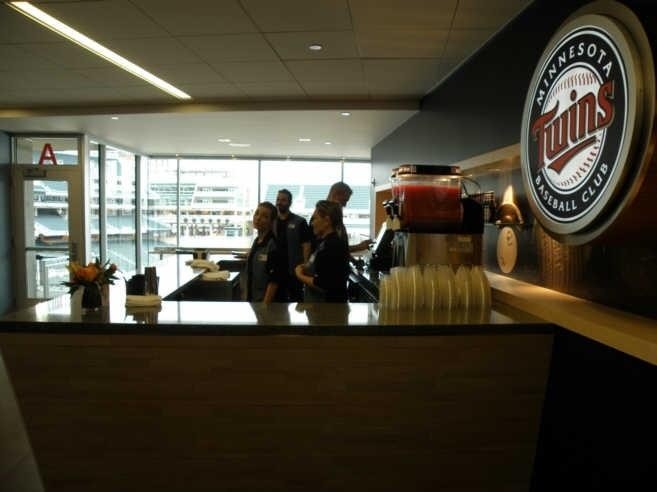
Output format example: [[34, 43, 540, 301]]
[[58, 256, 123, 298]]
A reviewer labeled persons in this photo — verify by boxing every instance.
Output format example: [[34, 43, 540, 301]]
[[270, 189, 310, 299], [295, 201, 349, 303], [246, 202, 280, 301], [309, 182, 372, 252]]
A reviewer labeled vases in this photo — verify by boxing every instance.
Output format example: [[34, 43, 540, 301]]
[[82, 285, 101, 308]]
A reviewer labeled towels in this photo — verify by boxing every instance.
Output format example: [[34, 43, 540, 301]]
[[126, 294, 161, 307], [185, 259, 220, 272], [201, 270, 230, 281]]
